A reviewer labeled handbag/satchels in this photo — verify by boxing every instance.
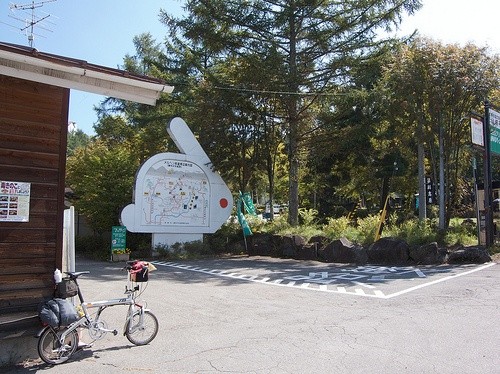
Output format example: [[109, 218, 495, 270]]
[[40, 298, 78, 326], [55, 277, 78, 298]]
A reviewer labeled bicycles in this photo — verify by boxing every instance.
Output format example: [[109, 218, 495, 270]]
[[34, 260, 159, 364]]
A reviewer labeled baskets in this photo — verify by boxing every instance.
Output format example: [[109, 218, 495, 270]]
[[130, 267, 148, 282]]
